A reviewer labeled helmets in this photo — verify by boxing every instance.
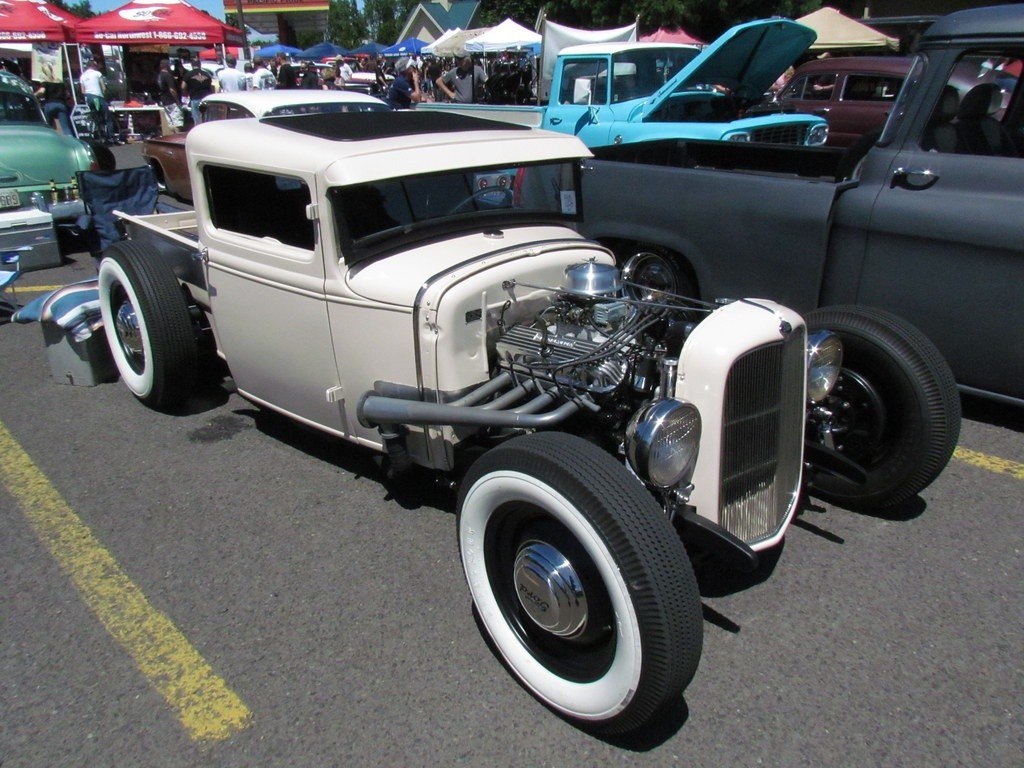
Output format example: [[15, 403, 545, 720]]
[[396, 58, 418, 74]]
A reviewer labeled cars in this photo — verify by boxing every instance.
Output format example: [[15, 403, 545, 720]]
[[96, 109, 961, 741], [0, 69, 102, 272], [142, 88, 393, 205]]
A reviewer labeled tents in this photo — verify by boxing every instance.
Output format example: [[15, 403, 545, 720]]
[[76, 0, 244, 94], [0, 0, 88, 116], [794, 6, 903, 54], [640, 23, 704, 48]]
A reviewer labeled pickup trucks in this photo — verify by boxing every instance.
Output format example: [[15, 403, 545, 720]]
[[408, 14, 831, 151], [571, 0, 1023, 428]]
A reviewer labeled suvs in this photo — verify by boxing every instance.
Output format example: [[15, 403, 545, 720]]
[[770, 55, 1019, 155]]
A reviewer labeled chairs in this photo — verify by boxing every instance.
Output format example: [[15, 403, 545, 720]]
[[956, 81, 1014, 155], [922, 83, 961, 155]]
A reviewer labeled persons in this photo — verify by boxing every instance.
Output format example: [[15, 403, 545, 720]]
[[156, 15, 543, 111], [31, 62, 76, 139], [80, 60, 122, 146]]
